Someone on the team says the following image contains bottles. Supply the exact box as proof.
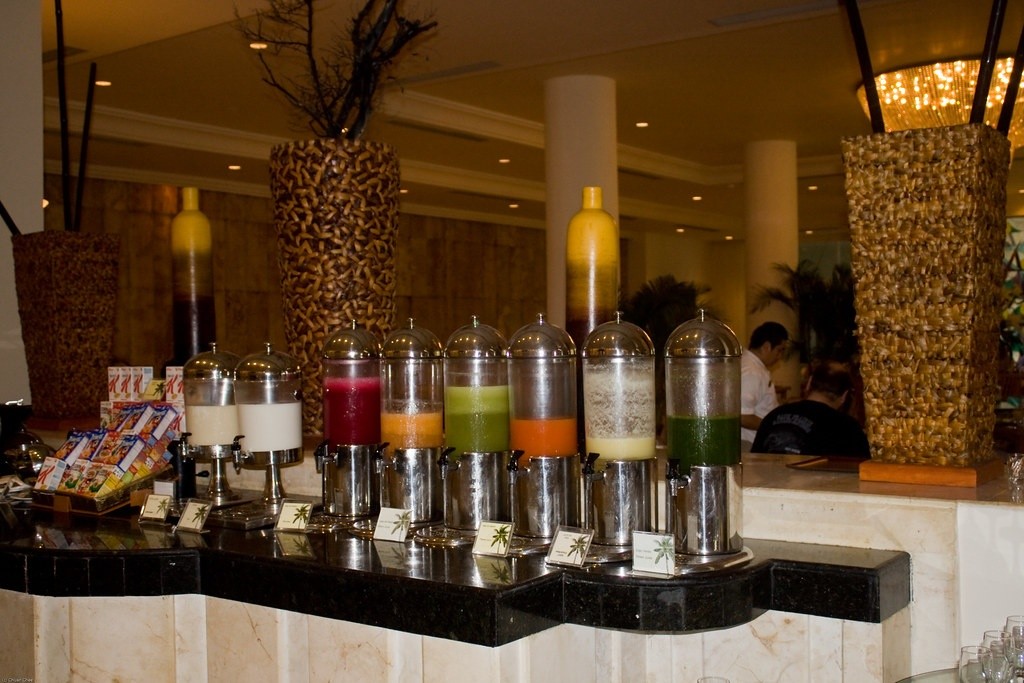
[565,186,620,466]
[170,187,215,365]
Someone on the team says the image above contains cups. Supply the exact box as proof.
[956,614,1024,683]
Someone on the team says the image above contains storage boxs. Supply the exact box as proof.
[35,367,186,497]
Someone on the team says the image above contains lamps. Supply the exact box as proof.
[855,58,1024,169]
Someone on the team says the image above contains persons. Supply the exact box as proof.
[749,358,872,458]
[740,321,789,445]
